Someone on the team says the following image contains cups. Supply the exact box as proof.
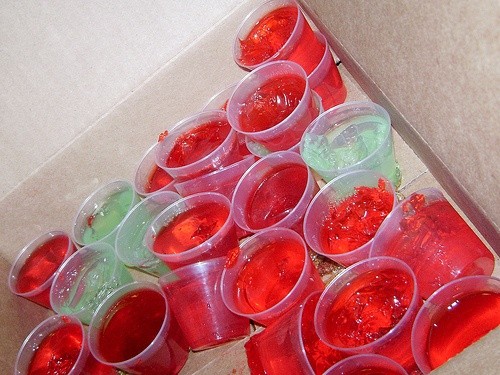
[87,281,189,375]
[13,313,119,375]
[245,88,326,159]
[49,242,137,328]
[172,154,258,238]
[224,60,319,154]
[115,189,188,282]
[231,150,324,262]
[145,193,241,280]
[321,353,410,375]
[233,0,323,79]
[131,141,182,197]
[220,229,324,328]
[367,186,495,306]
[306,30,347,113]
[153,110,244,181]
[302,169,404,268]
[411,273,500,375]
[71,180,144,257]
[155,257,248,354]
[314,255,424,375]
[197,84,246,147]
[244,292,345,375]
[7,230,85,313]
[299,99,401,194]
